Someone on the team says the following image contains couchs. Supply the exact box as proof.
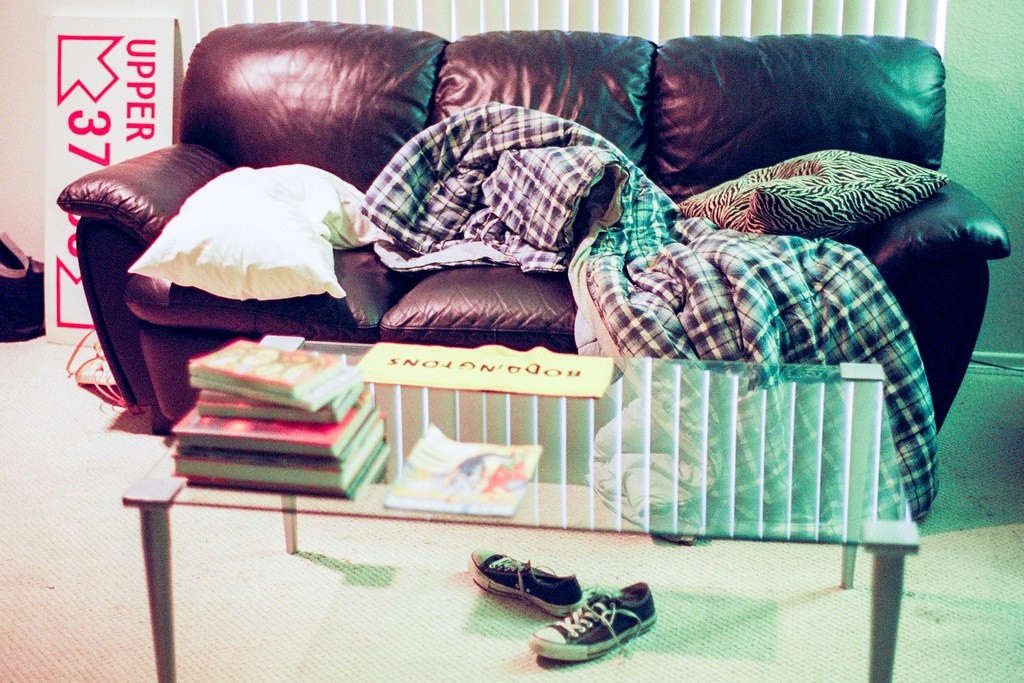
[56,20,1011,472]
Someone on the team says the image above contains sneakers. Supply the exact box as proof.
[528,582,657,661]
[467,548,583,617]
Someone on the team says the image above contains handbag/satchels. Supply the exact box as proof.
[0,232,45,342]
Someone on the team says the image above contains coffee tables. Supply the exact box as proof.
[125,334,921,681]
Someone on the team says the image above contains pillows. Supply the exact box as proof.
[679,149,949,232]
[129,163,371,302]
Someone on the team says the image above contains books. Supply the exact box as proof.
[171,338,390,502]
[383,422,544,518]
